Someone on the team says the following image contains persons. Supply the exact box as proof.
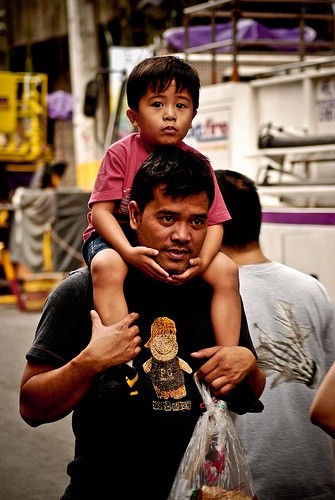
[209,170,335,499]
[310,359,335,439]
[19,145,267,500]
[81,56,265,416]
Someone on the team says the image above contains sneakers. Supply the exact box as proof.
[98,362,139,396]
[226,377,264,415]
